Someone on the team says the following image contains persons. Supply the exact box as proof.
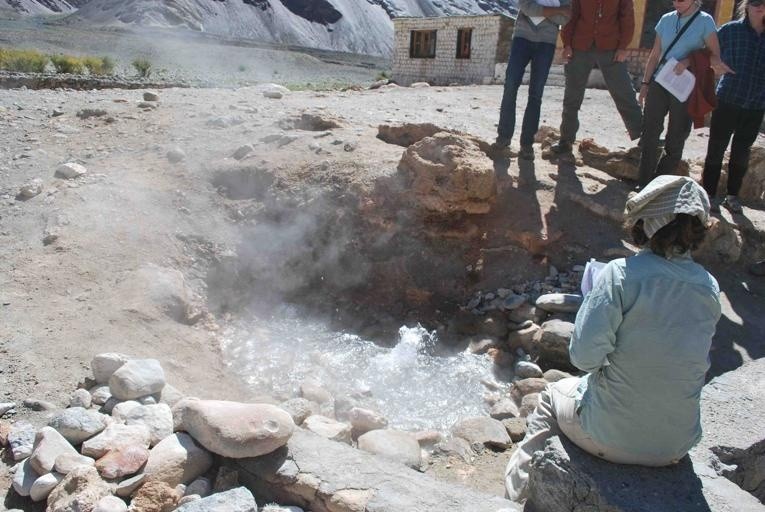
[550,1,667,153]
[504,174,724,504]
[635,1,721,193]
[699,1,764,214]
[494,1,574,159]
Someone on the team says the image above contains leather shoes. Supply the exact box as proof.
[634,184,646,191]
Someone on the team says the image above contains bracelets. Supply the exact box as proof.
[641,81,649,85]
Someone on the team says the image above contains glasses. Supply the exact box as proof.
[751,1,765,6]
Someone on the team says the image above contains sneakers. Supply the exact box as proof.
[550,141,572,152]
[496,137,510,147]
[522,134,534,144]
[710,198,720,213]
[722,198,742,215]
